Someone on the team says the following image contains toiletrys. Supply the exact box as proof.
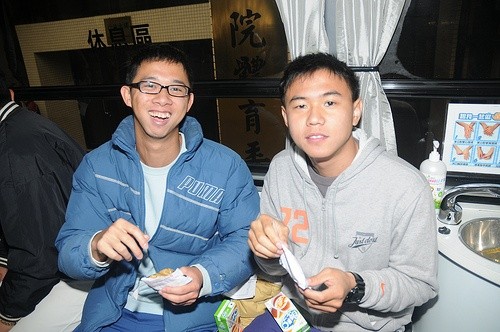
[420,140,447,215]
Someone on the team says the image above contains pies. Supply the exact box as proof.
[150,268,174,278]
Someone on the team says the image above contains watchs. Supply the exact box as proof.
[342,272,366,305]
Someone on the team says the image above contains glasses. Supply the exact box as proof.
[129,80,193,97]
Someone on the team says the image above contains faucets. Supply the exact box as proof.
[437,183,500,226]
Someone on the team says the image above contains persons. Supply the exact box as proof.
[0,78,86,331]
[243,51,439,332]
[54,42,261,332]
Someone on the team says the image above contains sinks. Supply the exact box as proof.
[438,208,500,287]
